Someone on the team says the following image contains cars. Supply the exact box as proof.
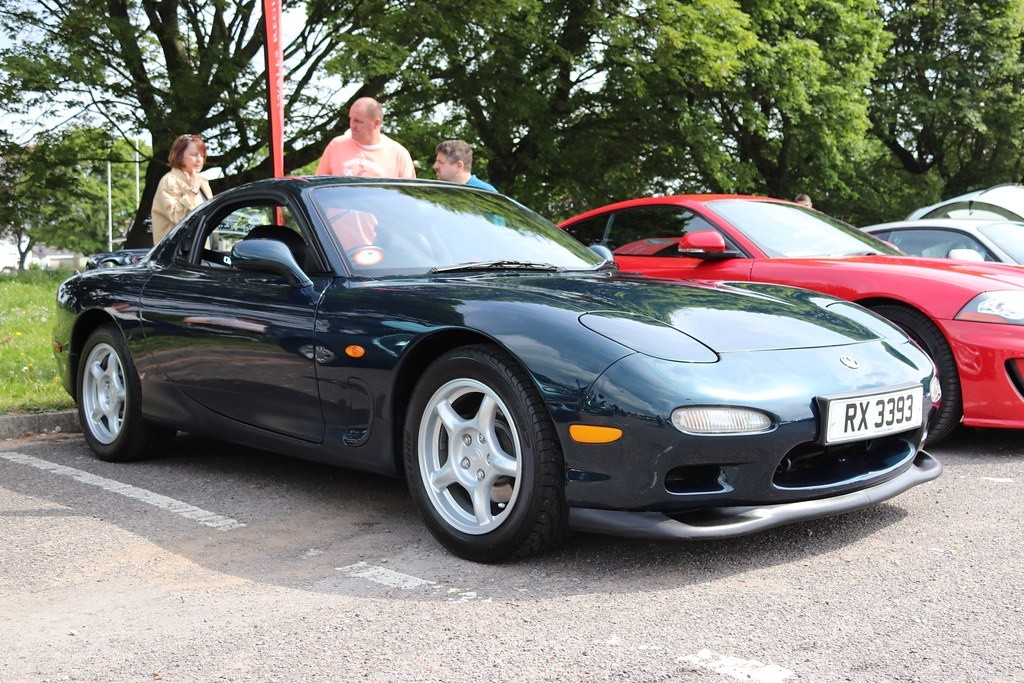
[50,176,943,566]
[555,195,1024,448]
[854,219,1024,266]
[903,184,1024,222]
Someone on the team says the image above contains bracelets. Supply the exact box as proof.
[188,186,199,195]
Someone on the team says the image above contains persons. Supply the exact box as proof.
[796,194,812,208]
[151,134,219,250]
[433,139,505,227]
[315,97,416,252]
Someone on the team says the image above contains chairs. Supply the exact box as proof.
[244,224,314,269]
[899,240,923,258]
